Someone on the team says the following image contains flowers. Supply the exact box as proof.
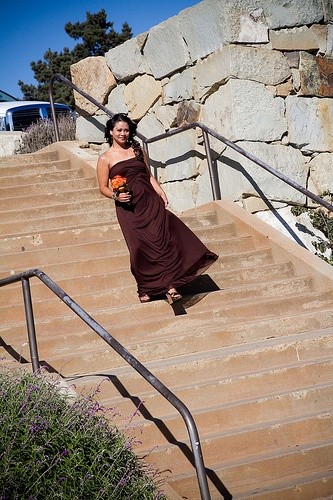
[111,175,132,207]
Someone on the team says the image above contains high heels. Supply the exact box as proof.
[140,295,150,303]
[165,292,182,304]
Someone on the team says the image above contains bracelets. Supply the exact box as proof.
[112,192,118,201]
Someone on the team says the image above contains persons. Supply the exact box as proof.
[97,113,219,304]
